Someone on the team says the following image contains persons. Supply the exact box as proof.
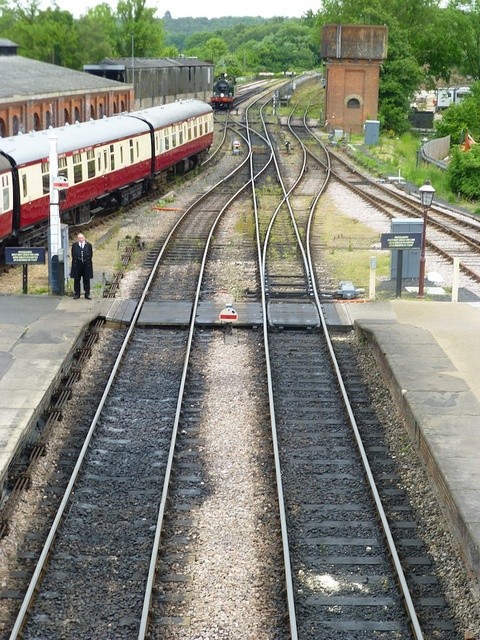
[70,234,93,300]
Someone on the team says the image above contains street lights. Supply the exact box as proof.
[418,178,435,297]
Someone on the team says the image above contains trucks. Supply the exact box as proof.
[437,91,472,111]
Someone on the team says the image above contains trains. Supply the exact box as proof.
[0,99,214,253]
[211,73,234,108]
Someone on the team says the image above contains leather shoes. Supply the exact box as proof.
[73,292,80,299]
[85,294,92,300]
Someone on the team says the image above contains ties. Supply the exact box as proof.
[80,243,83,262]
